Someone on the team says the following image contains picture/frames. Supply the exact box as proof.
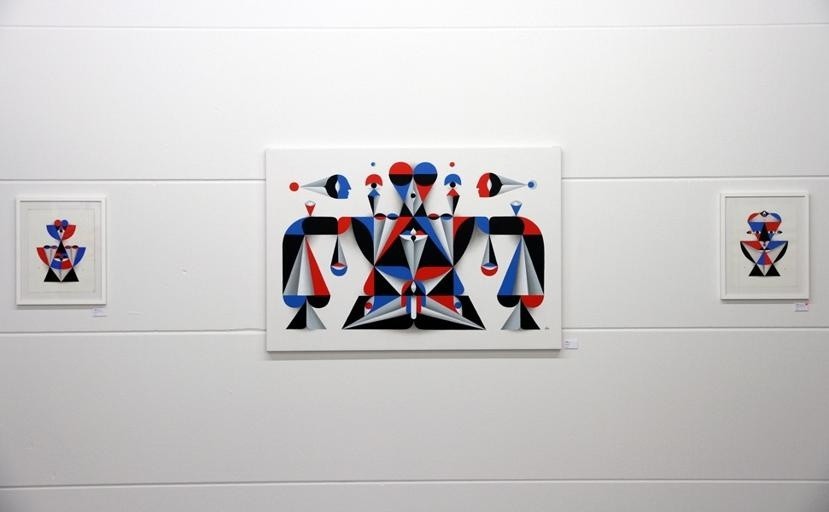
[719,192,812,302]
[16,196,108,306]
